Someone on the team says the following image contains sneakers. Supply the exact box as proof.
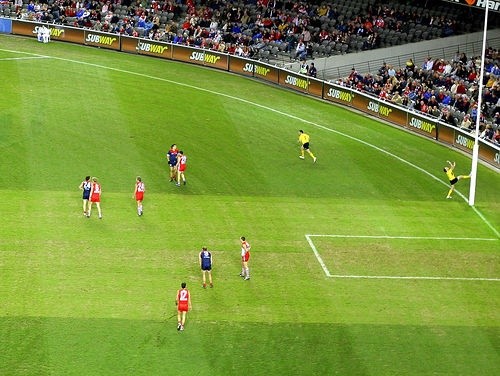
[240,274,250,282]
[299,155,305,160]
[446,196,452,199]
[168,177,187,187]
[138,210,143,216]
[177,324,184,331]
[313,157,317,164]
[82,210,101,219]
[203,283,214,291]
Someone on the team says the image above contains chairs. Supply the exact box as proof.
[0,0,500,136]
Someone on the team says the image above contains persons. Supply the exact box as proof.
[239,236,251,280]
[132,176,146,216]
[199,248,213,288]
[444,160,471,198]
[167,144,187,187]
[79,176,102,219]
[297,130,317,163]
[176,282,193,330]
[0,0,500,146]
